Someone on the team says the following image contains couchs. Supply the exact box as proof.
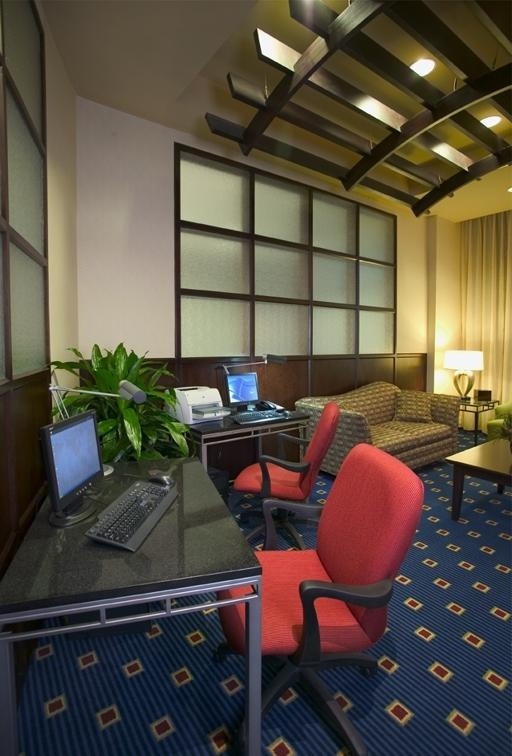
[294,380,459,479]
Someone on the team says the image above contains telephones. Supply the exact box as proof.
[255,399,286,412]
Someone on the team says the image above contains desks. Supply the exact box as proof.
[0,454,264,755]
[182,408,310,474]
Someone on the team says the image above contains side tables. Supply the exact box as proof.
[459,400,501,444]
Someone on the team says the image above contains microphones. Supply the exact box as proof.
[118,380,146,404]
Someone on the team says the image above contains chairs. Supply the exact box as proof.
[213,444,425,756]
[227,402,339,550]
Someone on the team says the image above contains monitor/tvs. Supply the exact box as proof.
[224,372,261,415]
[39,408,105,528]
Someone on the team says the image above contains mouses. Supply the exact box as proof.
[283,410,294,417]
[147,468,174,485]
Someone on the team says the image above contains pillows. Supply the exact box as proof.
[394,390,433,422]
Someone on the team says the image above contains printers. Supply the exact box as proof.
[163,386,231,425]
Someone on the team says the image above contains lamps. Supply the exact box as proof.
[443,351,485,401]
[46,380,147,476]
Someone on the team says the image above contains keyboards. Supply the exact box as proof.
[84,481,179,552]
[230,410,285,425]
